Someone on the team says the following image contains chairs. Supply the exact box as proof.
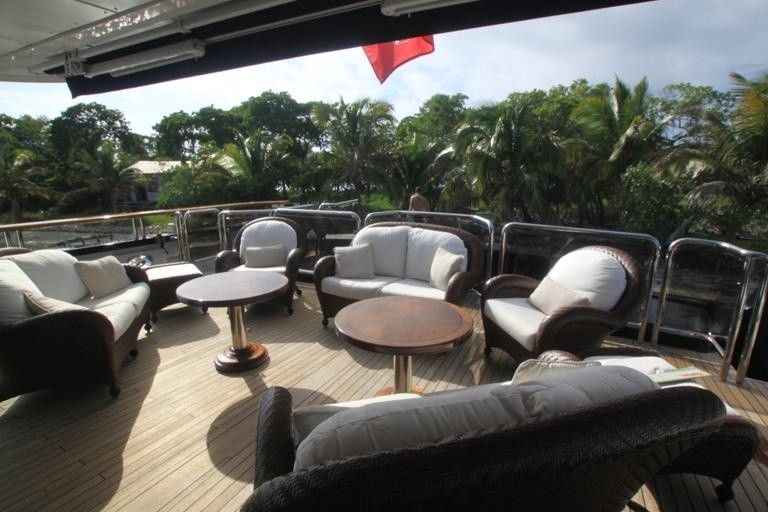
[481,245,647,365]
[215,217,306,315]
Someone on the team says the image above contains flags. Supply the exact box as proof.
[360,34,436,87]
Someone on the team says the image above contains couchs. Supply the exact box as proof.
[313,221,485,356]
[0,246,152,399]
[240,350,726,512]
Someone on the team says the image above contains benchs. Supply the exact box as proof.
[578,343,759,501]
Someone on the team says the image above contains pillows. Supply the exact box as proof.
[430,247,464,291]
[74,255,133,300]
[1,259,45,325]
[244,244,285,267]
[332,243,375,278]
[353,226,411,279]
[23,291,89,315]
[526,276,590,315]
[405,228,468,282]
[527,250,627,315]
[293,347,660,473]
[239,220,298,265]
[0,249,90,303]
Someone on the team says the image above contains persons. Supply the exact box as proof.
[408,186,426,223]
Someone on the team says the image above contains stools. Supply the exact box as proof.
[145,261,208,324]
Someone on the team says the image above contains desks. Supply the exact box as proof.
[175,271,288,372]
